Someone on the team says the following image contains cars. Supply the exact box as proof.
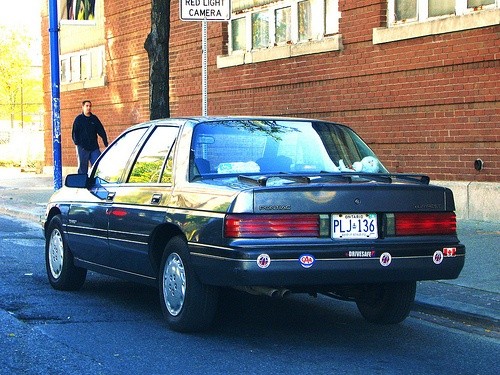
[41,114,466,334]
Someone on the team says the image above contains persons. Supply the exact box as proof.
[72,100,108,174]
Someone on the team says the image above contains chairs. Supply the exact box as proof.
[194,156,210,174]
[255,155,294,172]
